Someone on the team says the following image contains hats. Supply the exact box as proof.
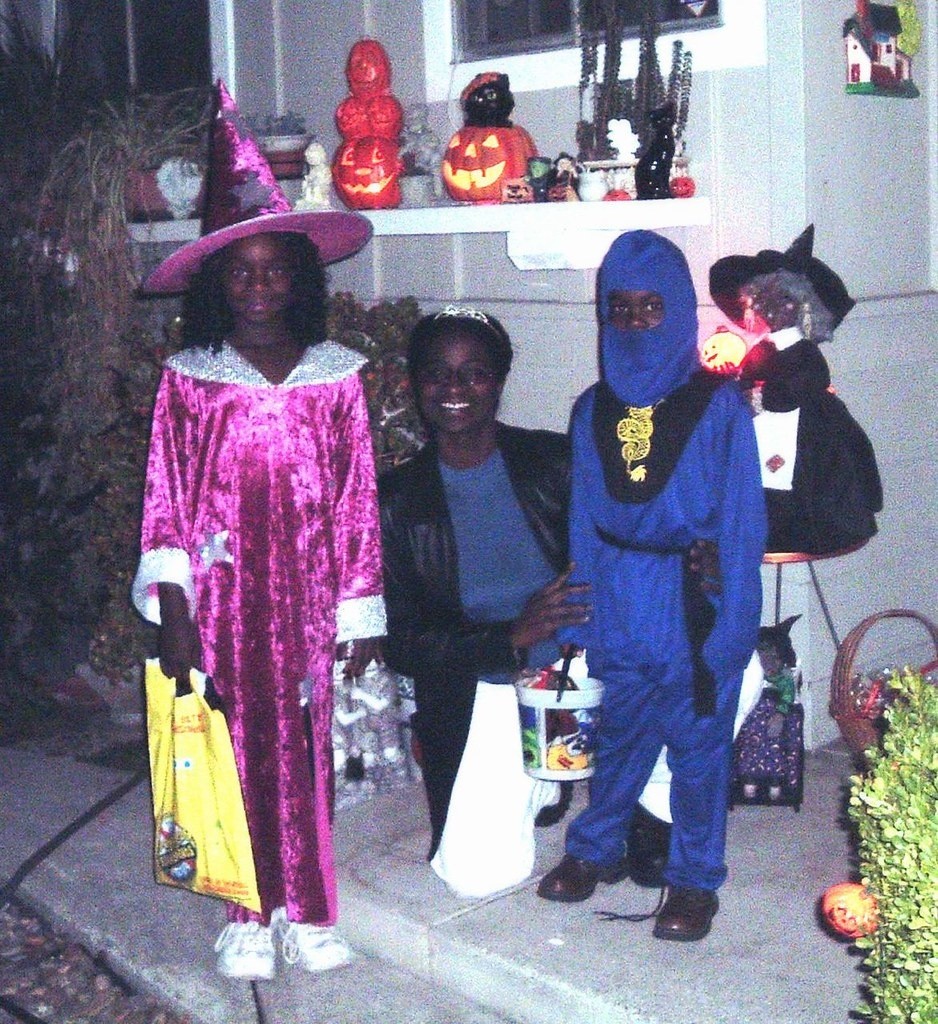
[138,80,375,294]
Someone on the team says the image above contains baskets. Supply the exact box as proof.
[830,608,938,774]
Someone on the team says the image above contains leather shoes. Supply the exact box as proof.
[537,853,625,901]
[653,885,717,937]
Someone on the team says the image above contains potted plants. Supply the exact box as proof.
[0,8,316,495]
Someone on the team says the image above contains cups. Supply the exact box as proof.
[276,179,305,208]
[265,152,309,178]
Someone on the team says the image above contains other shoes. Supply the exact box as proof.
[536,782,574,826]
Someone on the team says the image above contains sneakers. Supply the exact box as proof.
[214,920,275,981]
[276,915,355,972]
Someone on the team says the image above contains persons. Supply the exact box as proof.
[378,311,673,889]
[538,231,768,941]
[296,144,332,207]
[130,78,388,979]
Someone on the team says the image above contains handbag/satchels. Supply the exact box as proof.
[144,655,262,915]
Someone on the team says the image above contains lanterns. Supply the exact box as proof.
[442,121,536,199]
[670,176,695,199]
[331,136,401,210]
[701,326,745,372]
[822,883,879,937]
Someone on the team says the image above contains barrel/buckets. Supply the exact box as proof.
[516,647,607,781]
